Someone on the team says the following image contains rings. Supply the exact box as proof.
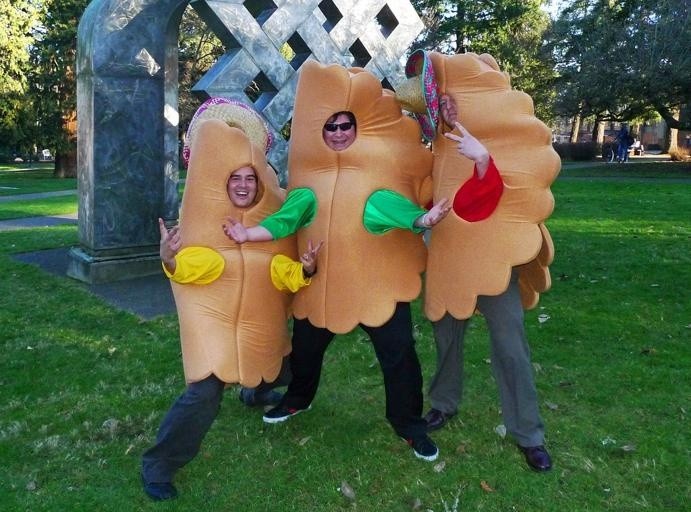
[306,255,309,260]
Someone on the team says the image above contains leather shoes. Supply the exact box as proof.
[516,441,553,472]
[420,406,458,428]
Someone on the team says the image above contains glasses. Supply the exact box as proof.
[322,121,353,131]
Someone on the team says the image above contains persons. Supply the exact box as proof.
[617,123,630,163]
[220,60,453,462]
[136,118,288,500]
[418,48,561,471]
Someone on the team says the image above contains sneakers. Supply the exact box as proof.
[141,469,179,501]
[261,401,313,424]
[238,388,284,406]
[400,432,439,462]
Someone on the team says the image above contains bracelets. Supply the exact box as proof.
[423,214,432,228]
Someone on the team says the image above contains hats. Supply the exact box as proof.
[393,47,439,143]
[182,95,272,173]
